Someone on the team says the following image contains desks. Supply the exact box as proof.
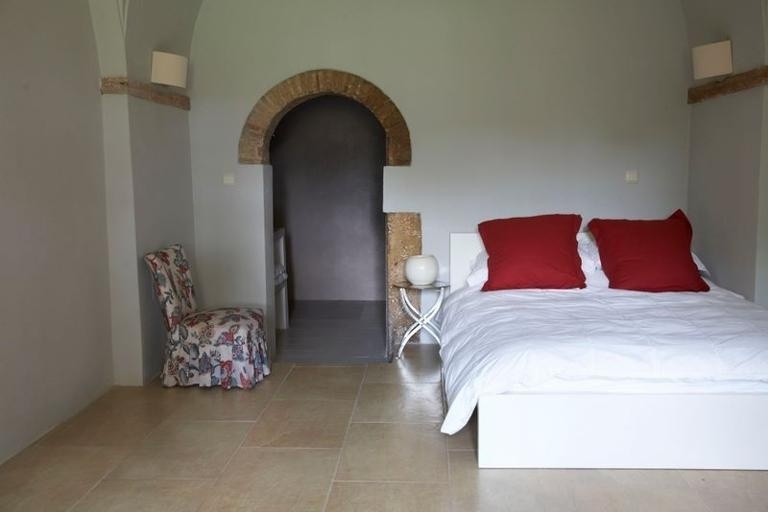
[393,281,451,361]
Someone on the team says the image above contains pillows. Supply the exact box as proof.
[466,210,711,292]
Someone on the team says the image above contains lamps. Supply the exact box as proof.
[150,51,188,89]
[692,39,733,81]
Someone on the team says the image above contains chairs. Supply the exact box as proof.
[144,244,270,389]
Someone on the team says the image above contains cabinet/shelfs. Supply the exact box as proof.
[274,227,290,331]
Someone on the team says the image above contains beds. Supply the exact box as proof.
[449,232,768,470]
